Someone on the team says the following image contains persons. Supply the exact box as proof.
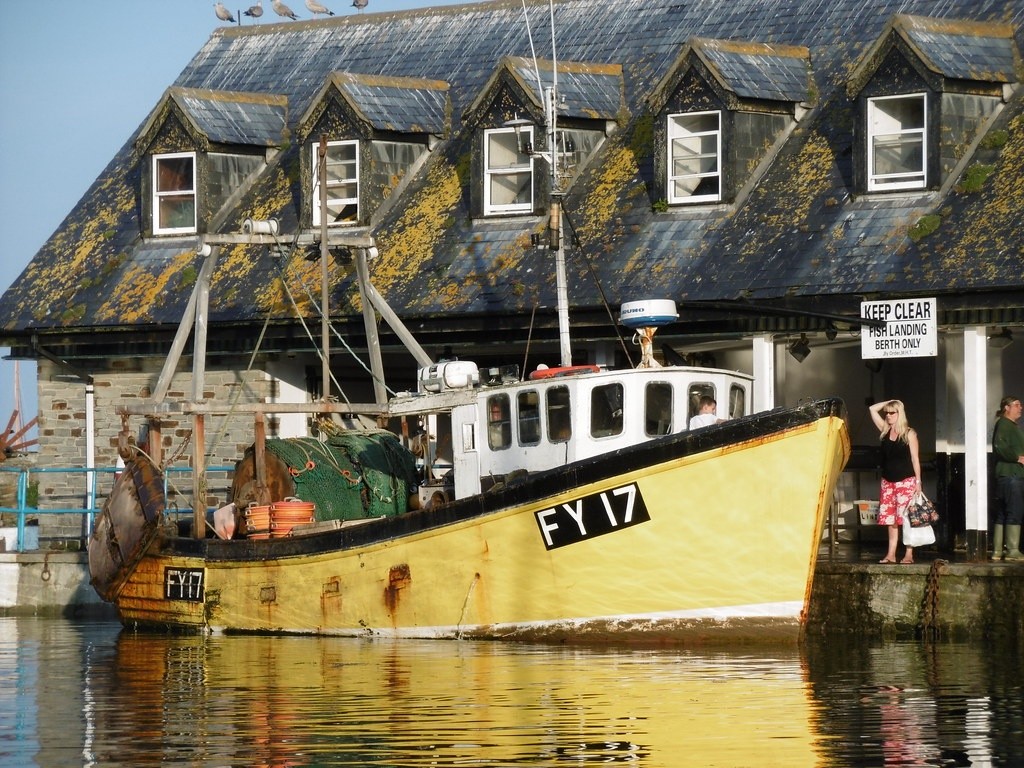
[991,396,1024,561]
[878,684,938,767]
[868,399,922,564]
[689,396,730,431]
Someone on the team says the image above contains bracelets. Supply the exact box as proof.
[916,480,922,484]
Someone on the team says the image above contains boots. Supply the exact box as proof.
[1004,525,1024,561]
[991,524,1006,560]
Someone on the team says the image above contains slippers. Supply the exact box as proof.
[878,559,897,564]
[900,559,916,564]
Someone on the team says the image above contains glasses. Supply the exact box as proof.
[885,411,898,415]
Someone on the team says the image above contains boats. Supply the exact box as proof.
[111,1,850,635]
[115,630,829,768]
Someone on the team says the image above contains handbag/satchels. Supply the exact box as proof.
[903,491,939,547]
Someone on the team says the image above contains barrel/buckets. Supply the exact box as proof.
[244,497,316,540]
